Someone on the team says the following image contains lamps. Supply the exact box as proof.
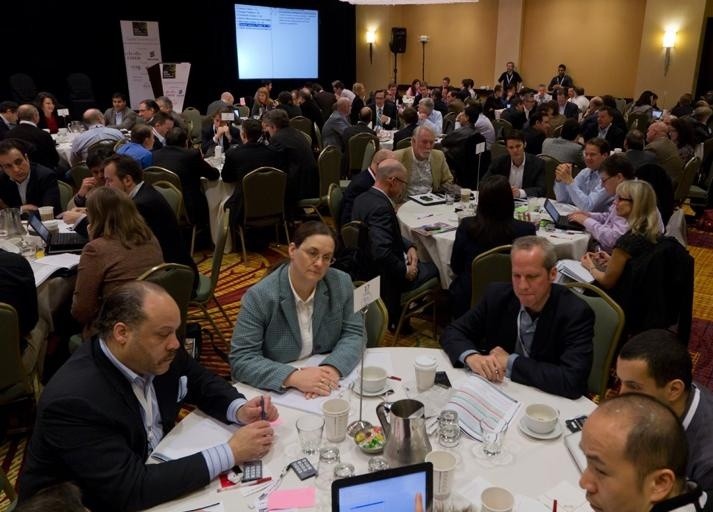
[419,35,429,83]
[662,31,674,77]
[366,32,378,65]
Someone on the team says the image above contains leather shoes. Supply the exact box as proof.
[387,322,415,337]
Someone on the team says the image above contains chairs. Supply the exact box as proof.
[564,283,622,397]
[2,304,36,502]
[473,244,516,309]
[355,282,388,347]
[66,263,199,358]
[1,98,713,257]
[192,208,235,346]
[338,220,441,345]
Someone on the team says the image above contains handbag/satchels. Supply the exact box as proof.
[180,322,231,383]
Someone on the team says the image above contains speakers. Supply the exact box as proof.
[389,27,407,53]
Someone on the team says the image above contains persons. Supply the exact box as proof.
[0,62,713,404]
[414,493,423,512]
[617,327,713,495]
[13,279,278,512]
[13,481,90,512]
[578,391,713,511]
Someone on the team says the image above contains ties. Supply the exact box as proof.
[377,109,381,127]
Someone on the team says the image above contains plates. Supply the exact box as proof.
[352,383,391,397]
[518,415,565,440]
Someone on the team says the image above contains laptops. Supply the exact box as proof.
[331,462,434,511]
[29,213,88,252]
[544,200,586,231]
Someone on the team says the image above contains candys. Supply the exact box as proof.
[357,426,387,449]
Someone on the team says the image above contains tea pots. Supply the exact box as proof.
[376,399,432,466]
[0,207,27,239]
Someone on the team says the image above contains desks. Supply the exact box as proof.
[142,346,596,509]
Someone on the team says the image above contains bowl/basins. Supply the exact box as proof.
[355,426,386,454]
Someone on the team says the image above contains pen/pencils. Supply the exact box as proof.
[553,499,557,512]
[495,370,499,374]
[550,235,573,240]
[387,376,401,381]
[217,465,290,499]
[260,395,265,420]
[417,214,434,219]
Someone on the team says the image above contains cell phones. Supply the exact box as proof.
[433,372,452,390]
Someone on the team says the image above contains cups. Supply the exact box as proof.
[368,457,389,474]
[296,415,324,454]
[322,398,351,442]
[480,415,508,456]
[37,205,54,221]
[461,188,471,203]
[21,240,37,263]
[457,208,474,224]
[358,366,387,392]
[439,410,460,448]
[413,355,440,391]
[424,450,457,500]
[480,486,514,512]
[527,196,537,211]
[525,403,561,434]
[315,447,355,491]
[445,192,455,206]
[67,121,81,133]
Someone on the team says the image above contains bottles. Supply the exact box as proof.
[215,146,220,158]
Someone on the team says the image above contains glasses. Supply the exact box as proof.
[300,247,335,266]
[601,174,613,185]
[613,194,632,204]
[389,176,409,187]
[319,447,355,479]
